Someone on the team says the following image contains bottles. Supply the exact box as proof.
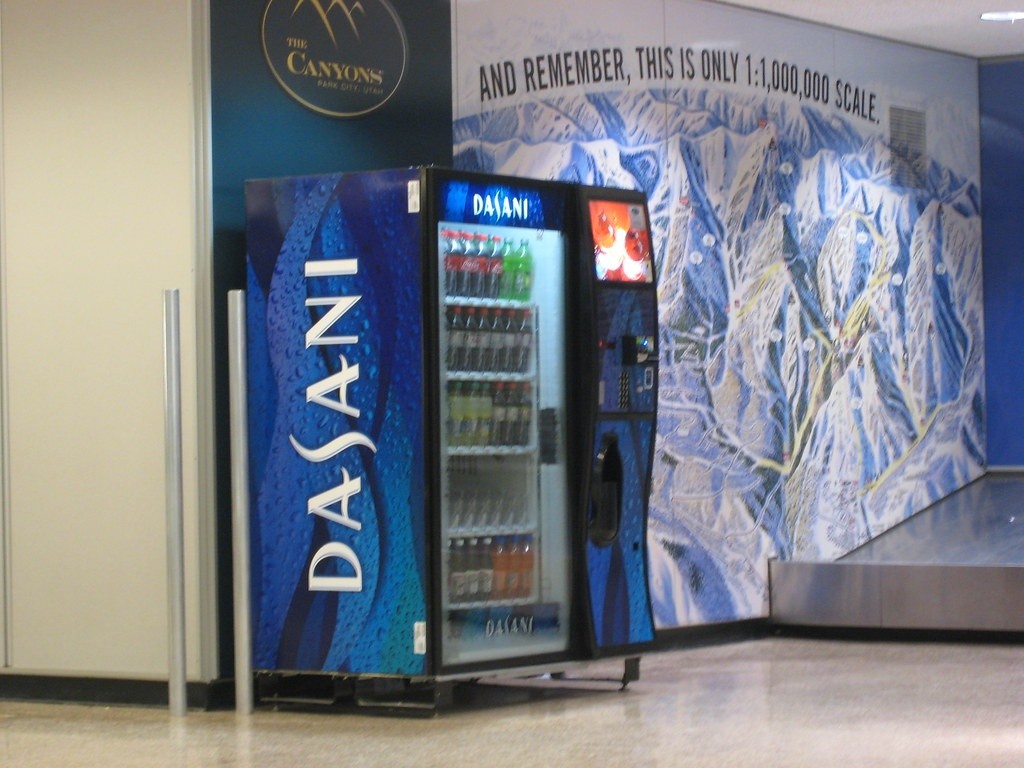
[446,306,532,373]
[448,535,536,604]
[441,228,532,302]
[448,489,530,530]
[447,380,533,446]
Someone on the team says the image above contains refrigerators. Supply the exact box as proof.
[242,164,659,718]
